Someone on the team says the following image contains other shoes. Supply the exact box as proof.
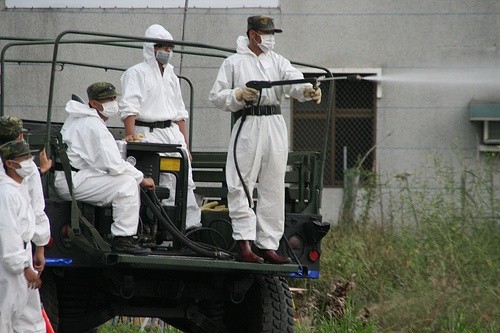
[112,236,152,256]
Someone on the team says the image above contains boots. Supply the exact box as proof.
[251,241,293,264]
[235,240,264,264]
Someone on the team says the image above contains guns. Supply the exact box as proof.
[245,75,361,107]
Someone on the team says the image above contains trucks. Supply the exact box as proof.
[0,30,336,332]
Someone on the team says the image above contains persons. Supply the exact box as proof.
[55,82,155,256]
[209,16,321,264]
[0,115,52,333]
[119,24,202,230]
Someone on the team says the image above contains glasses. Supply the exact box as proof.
[154,43,175,49]
[14,155,32,161]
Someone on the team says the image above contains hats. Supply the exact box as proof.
[87,82,122,100]
[0,140,40,160]
[247,16,283,33]
[0,115,28,135]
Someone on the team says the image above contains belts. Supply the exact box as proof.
[236,104,282,117]
[135,119,172,132]
[54,161,81,172]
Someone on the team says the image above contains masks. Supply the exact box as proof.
[249,30,275,53]
[94,100,119,117]
[155,50,173,65]
[6,156,36,179]
